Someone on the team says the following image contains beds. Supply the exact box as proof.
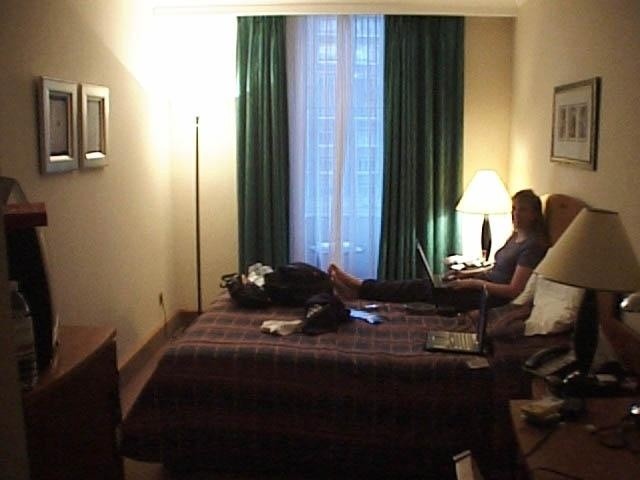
[122,194,640,466]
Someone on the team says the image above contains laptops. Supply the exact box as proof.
[424,282,488,355]
[417,241,482,292]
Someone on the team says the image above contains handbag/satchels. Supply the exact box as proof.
[220,272,264,307]
[302,295,344,334]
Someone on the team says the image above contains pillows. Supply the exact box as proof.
[510,247,553,310]
[524,276,587,340]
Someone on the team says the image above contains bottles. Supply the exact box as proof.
[9,280,40,390]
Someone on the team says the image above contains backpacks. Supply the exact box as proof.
[264,262,332,304]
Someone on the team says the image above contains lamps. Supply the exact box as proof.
[531,206,640,397]
[453,169,513,264]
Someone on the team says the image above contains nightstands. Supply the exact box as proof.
[508,398,640,479]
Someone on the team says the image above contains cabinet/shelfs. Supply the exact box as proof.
[19,326,124,480]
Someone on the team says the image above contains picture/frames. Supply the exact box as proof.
[32,75,80,177]
[549,76,601,171]
[79,82,110,169]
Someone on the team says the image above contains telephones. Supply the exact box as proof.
[520,344,578,382]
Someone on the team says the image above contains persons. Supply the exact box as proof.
[326,187,551,309]
